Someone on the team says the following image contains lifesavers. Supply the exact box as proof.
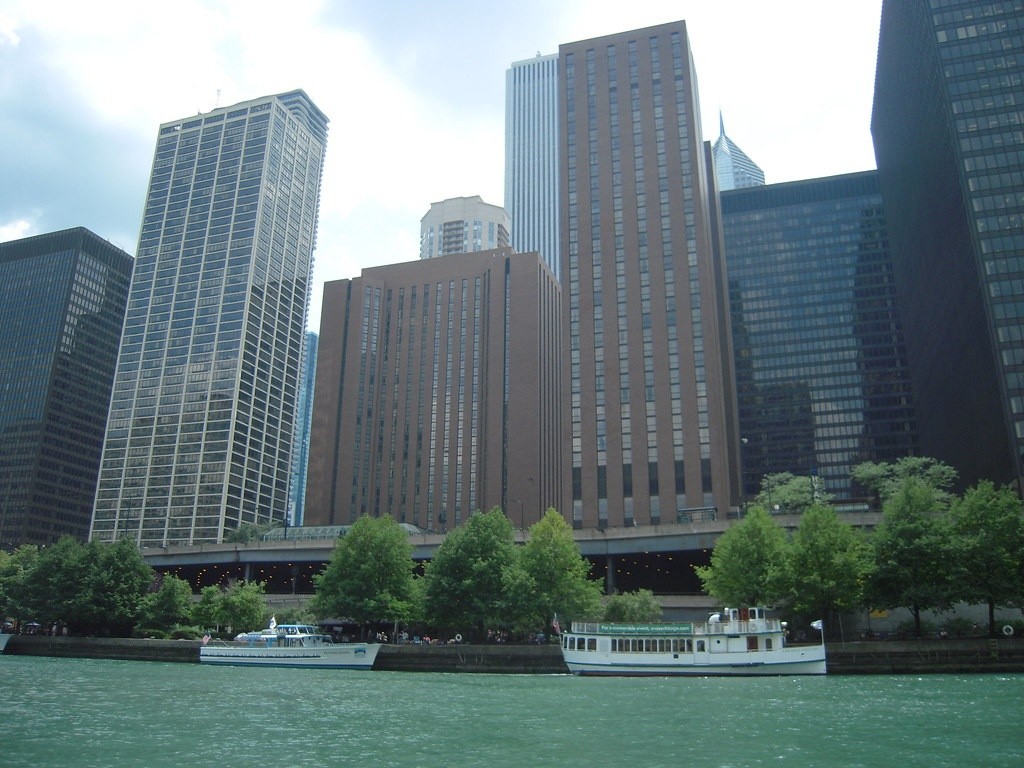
[1002,625,1013,636]
[455,634,462,641]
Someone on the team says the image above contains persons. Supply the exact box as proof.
[2,616,68,636]
[781,622,789,646]
[972,621,979,637]
[486,629,546,644]
[334,624,443,646]
[937,628,953,640]
[860,628,890,642]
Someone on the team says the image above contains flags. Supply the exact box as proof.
[552,613,560,637]
[810,620,822,630]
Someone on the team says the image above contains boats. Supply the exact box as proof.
[199,613,383,669]
[557,606,828,676]
